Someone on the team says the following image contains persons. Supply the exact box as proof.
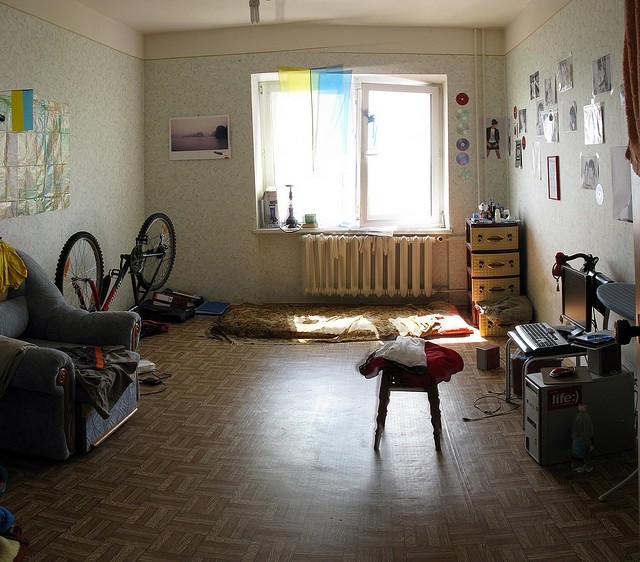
[588,159,598,189]
[529,76,538,99]
[536,103,544,136]
[568,106,576,132]
[486,118,501,159]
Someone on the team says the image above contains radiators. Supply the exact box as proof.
[300,232,436,298]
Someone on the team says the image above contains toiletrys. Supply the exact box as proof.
[495,209,501,223]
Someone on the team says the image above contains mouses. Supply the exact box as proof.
[549,367,576,378]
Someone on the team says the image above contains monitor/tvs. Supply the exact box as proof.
[556,265,591,343]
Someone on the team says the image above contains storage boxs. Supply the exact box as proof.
[474,301,529,337]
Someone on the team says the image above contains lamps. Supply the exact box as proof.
[249,0,260,24]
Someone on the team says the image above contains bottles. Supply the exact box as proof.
[495,209,500,223]
[571,404,595,472]
[489,198,494,212]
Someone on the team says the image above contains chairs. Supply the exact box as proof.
[505,266,614,430]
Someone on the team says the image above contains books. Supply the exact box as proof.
[152,300,193,312]
[193,300,231,315]
[152,291,195,307]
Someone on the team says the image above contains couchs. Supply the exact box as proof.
[0,246,142,471]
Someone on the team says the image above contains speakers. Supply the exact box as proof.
[587,344,622,377]
[476,344,500,371]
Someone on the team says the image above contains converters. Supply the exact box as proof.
[144,377,160,386]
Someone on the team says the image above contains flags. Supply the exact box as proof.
[10,89,34,132]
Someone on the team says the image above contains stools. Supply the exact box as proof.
[374,368,444,453]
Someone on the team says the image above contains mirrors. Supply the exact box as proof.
[546,155,560,200]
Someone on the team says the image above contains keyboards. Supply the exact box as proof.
[514,322,570,351]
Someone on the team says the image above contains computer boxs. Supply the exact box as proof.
[524,369,634,466]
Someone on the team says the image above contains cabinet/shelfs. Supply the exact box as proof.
[463,216,527,328]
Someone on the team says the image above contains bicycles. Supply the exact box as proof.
[53,212,176,339]
